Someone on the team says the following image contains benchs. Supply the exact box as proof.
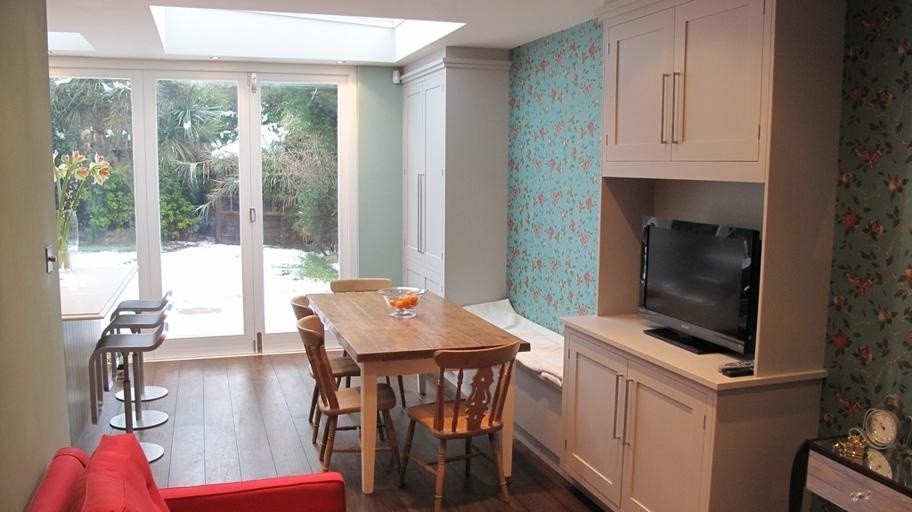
[459,298,566,474]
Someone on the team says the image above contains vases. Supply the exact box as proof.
[55,209,79,276]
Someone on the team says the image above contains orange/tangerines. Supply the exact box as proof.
[389,295,418,309]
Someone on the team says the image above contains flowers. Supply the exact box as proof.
[52,149,114,268]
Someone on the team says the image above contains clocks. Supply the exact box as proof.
[866,447,905,483]
[846,393,911,451]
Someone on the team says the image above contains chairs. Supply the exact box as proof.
[297,314,400,477]
[24,432,348,512]
[291,294,387,442]
[399,339,520,511]
[329,277,408,407]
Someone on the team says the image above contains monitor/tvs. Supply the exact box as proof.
[638,216,761,355]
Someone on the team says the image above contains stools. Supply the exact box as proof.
[88,321,170,462]
[110,290,174,402]
[101,302,173,429]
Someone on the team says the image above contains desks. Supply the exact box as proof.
[61,260,139,447]
[305,288,530,495]
[802,430,912,512]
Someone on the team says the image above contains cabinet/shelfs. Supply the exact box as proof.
[601,1,846,181]
[401,59,510,394]
[558,322,827,511]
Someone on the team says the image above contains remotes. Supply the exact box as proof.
[718,360,754,370]
[725,369,753,377]
